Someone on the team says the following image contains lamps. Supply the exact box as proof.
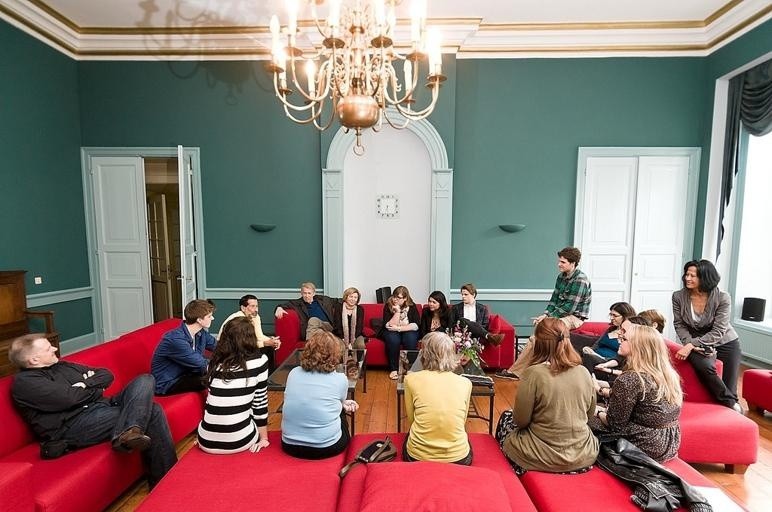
[266,0,444,158]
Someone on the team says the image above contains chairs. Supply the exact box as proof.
[0,270,61,378]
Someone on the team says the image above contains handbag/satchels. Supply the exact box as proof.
[356,440,398,463]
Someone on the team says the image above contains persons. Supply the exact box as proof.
[281,329,360,460]
[7,331,178,492]
[572,301,637,363]
[493,246,592,382]
[401,331,473,466]
[585,324,684,464]
[216,294,285,391]
[495,317,600,477]
[582,308,667,380]
[590,316,653,402]
[196,316,271,453]
[274,281,506,382]
[150,299,218,396]
[670,259,744,415]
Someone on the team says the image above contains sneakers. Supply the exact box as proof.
[389,372,399,380]
[112,427,151,454]
[486,333,504,346]
[495,369,519,381]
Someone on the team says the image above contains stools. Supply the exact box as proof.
[677,402,759,474]
[743,369,772,413]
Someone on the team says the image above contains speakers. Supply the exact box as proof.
[740,297,766,322]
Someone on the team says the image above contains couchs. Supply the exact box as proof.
[570,322,723,402]
[0,318,212,512]
[275,304,515,369]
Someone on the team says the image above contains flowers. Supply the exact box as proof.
[416,321,489,370]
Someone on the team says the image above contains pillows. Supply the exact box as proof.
[360,461,512,512]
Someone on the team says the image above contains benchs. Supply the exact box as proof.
[337,432,538,512]
[519,457,744,512]
[134,431,347,512]
